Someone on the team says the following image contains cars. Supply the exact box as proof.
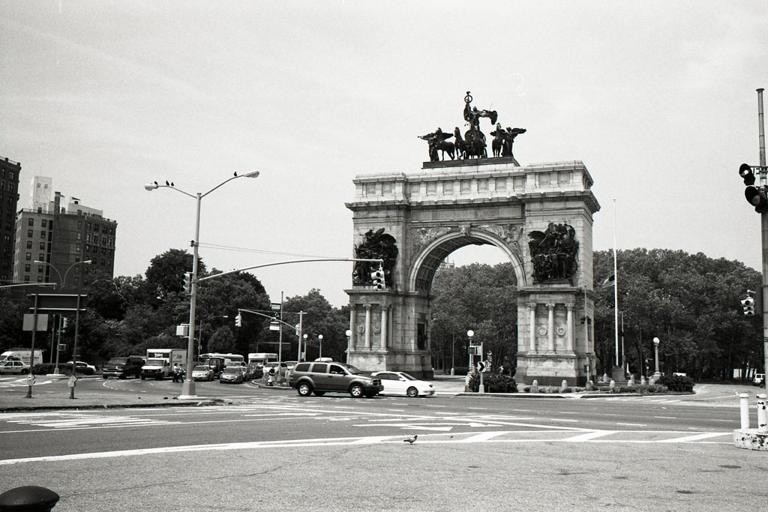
[369,371,435,398]
[0,360,31,374]
[192,352,298,384]
[66,360,96,375]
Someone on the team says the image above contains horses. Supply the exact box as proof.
[430,123,511,162]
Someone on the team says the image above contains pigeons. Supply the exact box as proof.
[404,435,418,445]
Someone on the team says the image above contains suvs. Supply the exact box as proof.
[289,361,384,398]
[102,357,145,379]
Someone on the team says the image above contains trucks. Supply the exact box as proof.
[139,348,187,380]
[0,348,47,368]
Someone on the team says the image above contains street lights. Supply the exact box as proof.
[346,330,352,364]
[144,170,259,400]
[197,315,228,363]
[303,334,308,362]
[467,329,474,377]
[34,260,91,374]
[653,337,661,380]
[318,334,323,362]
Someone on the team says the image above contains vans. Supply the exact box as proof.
[752,373,765,385]
[313,357,332,362]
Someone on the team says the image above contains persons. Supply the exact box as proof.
[173,363,179,383]
[268,365,275,386]
[178,363,184,383]
[417,102,526,163]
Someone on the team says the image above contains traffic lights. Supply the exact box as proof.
[182,273,190,295]
[235,314,241,327]
[741,297,755,318]
[376,270,385,291]
[743,184,766,214]
